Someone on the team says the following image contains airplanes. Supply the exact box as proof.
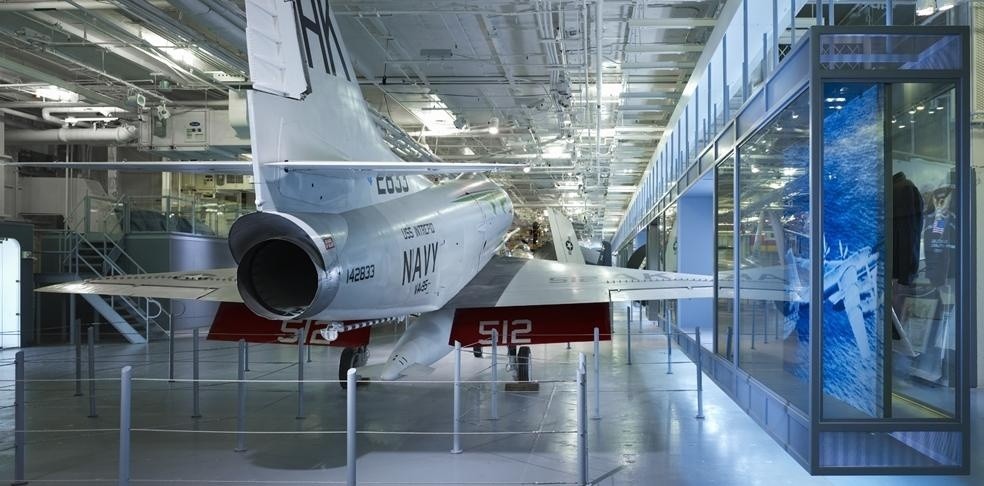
[0,0,716,388]
